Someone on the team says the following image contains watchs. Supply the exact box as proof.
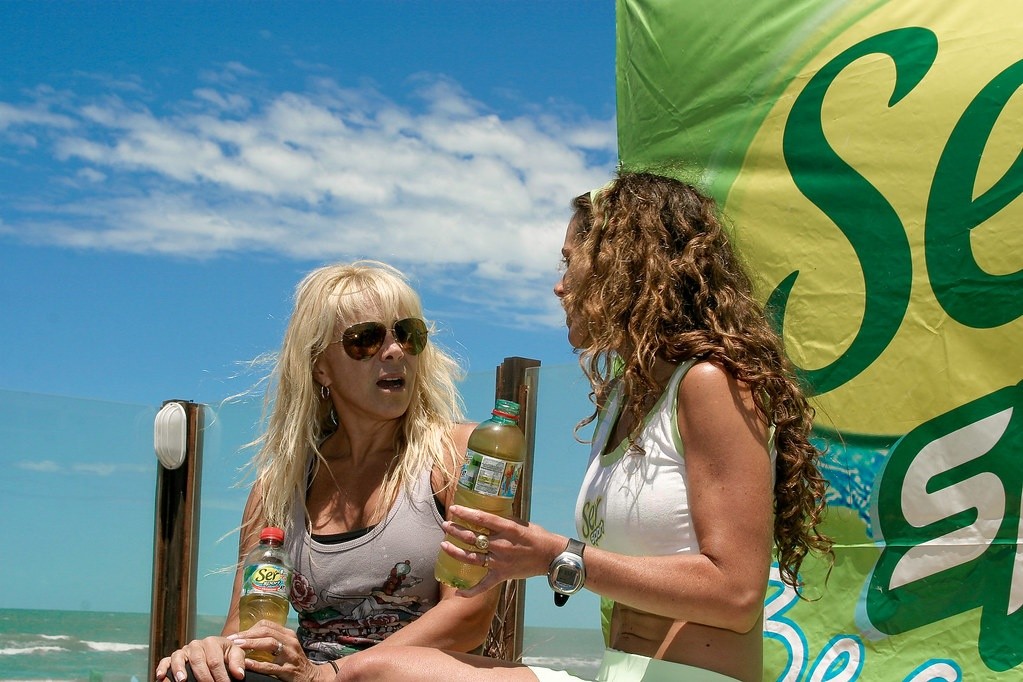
[548,538,586,607]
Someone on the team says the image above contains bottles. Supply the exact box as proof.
[238,527,293,665]
[434,399,527,590]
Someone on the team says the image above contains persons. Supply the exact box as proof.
[335,173,836,682]
[154,255,501,682]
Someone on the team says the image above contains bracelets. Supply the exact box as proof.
[327,660,339,674]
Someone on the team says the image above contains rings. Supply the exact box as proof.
[482,553,489,567]
[475,534,490,550]
[271,642,283,656]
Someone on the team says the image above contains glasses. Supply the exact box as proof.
[326,318,428,362]
